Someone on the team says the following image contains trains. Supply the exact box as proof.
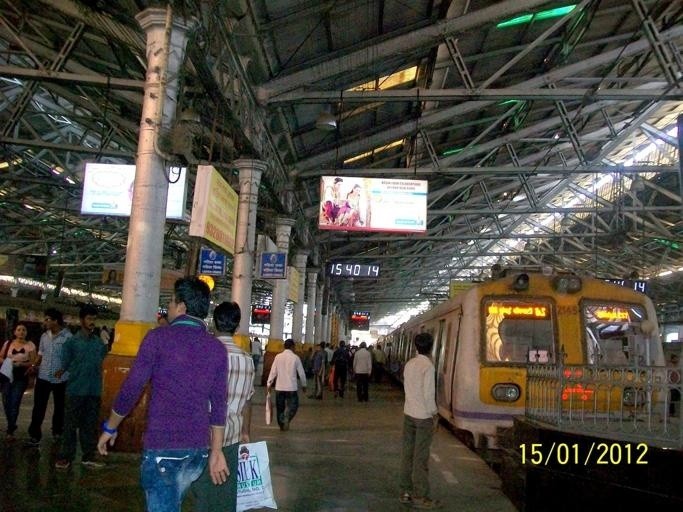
[0,294,123,342]
[370,260,671,474]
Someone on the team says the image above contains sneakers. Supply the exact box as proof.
[280,414,290,430]
[307,381,371,402]
[410,495,444,508]
[81,455,106,467]
[1,426,18,442]
[54,456,72,470]
[21,432,41,444]
[398,489,412,504]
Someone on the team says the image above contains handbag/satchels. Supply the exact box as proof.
[0,369,8,394]
[264,391,273,426]
[234,440,277,511]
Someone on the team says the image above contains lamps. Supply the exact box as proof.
[629,173,646,192]
[523,238,533,251]
[178,98,200,124]
[315,101,338,131]
[496,255,505,265]
[560,212,574,227]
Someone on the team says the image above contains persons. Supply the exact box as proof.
[322,177,344,225]
[96,276,230,511]
[338,185,366,228]
[187,301,257,512]
[399,331,446,509]
[666,355,683,420]
[2,304,119,470]
[254,331,394,428]
